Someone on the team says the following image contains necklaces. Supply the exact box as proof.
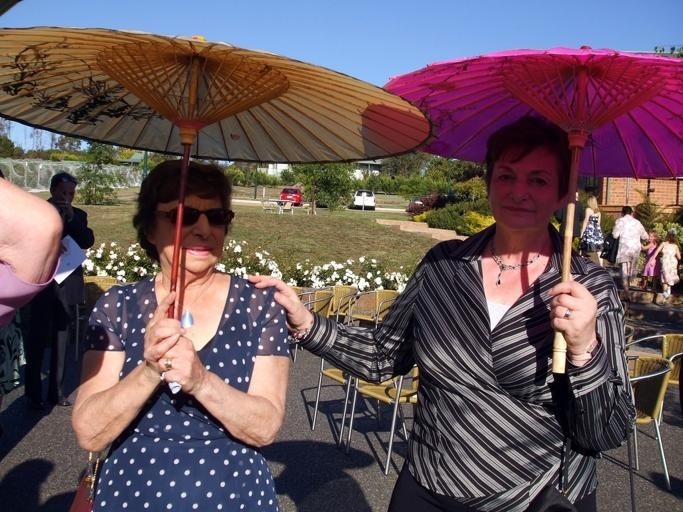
[489,234,551,289]
[159,269,224,330]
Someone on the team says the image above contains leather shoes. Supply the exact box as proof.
[55,399,70,406]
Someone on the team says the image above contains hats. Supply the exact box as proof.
[622,206,633,214]
[669,228,677,234]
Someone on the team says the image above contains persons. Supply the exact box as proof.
[245,112,641,512]
[610,204,649,302]
[18,170,96,414]
[637,229,661,292]
[70,158,301,512]
[651,231,682,306]
[0,175,65,328]
[577,193,606,269]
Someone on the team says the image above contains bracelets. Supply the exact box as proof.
[287,310,314,345]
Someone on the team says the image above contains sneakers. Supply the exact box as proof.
[666,289,671,294]
[663,292,669,299]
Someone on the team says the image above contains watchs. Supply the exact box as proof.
[567,339,600,363]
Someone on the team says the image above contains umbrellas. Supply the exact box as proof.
[381,42,683,377]
[1,22,441,339]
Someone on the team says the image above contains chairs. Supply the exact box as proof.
[622,324,683,493]
[260,196,293,215]
[63,274,120,364]
[288,282,419,474]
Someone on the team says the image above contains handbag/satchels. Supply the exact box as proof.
[70,465,97,512]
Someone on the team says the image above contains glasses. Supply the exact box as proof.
[157,207,234,226]
[59,175,77,184]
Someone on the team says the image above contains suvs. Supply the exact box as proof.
[279,187,302,206]
[352,189,375,208]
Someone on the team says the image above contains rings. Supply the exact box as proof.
[563,308,574,319]
[163,356,175,369]
